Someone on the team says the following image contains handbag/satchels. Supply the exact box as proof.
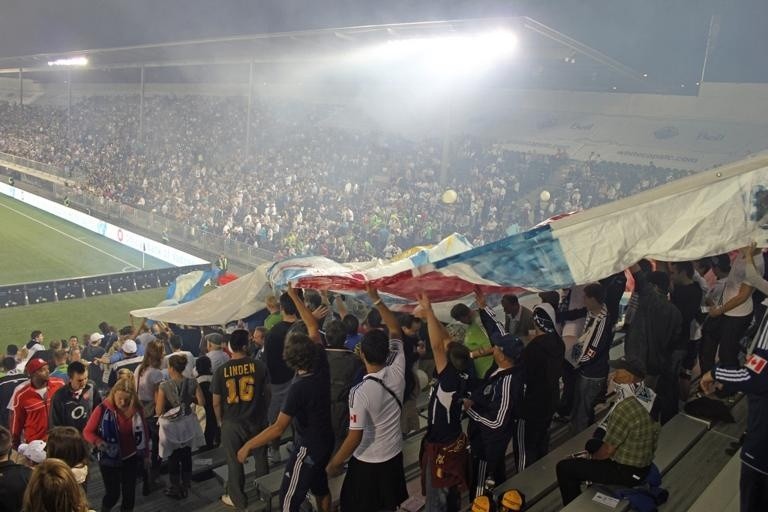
[89,425,104,462]
[143,402,157,424]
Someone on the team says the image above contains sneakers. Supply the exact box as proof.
[220,494,235,506]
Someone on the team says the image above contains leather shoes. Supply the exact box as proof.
[162,486,188,500]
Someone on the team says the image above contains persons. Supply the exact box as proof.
[0,92,768,511]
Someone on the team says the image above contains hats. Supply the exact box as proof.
[491,331,524,359]
[122,340,137,354]
[361,330,389,362]
[205,333,222,344]
[28,359,48,374]
[18,440,48,463]
[528,303,556,333]
[170,335,183,345]
[91,333,105,342]
[607,355,647,379]
